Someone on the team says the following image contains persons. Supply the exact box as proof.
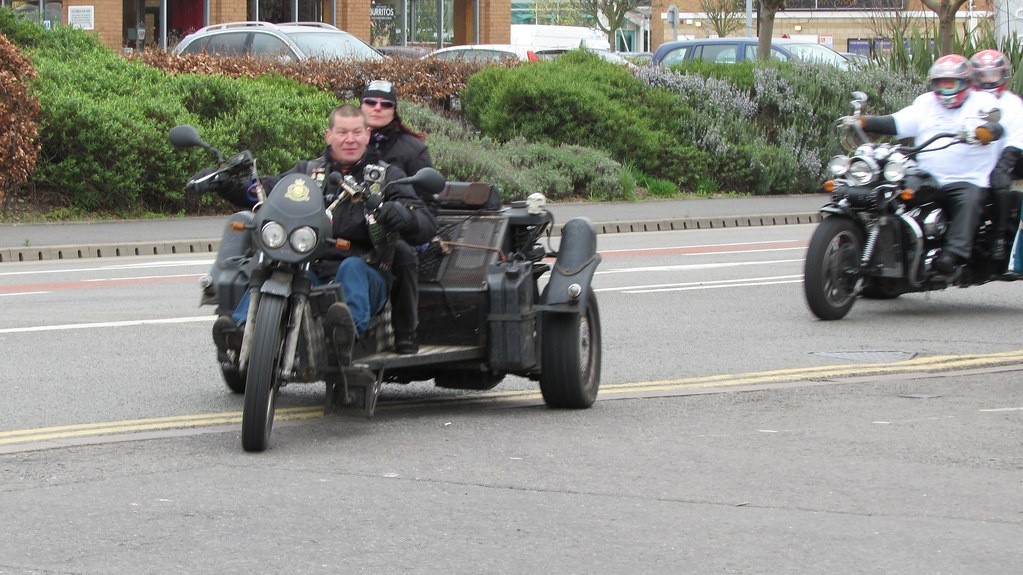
[841,53,1008,275]
[183,104,437,393]
[970,49,1023,261]
[358,79,433,354]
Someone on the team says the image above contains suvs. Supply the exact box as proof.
[164,21,393,100]
[651,36,863,74]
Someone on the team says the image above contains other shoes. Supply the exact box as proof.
[935,252,957,272]
[324,302,356,367]
[991,239,1007,260]
[212,315,238,363]
[396,332,419,353]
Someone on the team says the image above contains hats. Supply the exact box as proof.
[362,80,397,105]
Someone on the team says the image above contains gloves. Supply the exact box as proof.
[374,201,417,233]
[975,127,993,144]
[855,116,867,128]
[185,168,236,193]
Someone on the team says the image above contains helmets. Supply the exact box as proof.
[928,54,972,108]
[969,49,1011,99]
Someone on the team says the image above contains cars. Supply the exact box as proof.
[374,45,436,60]
[839,52,884,70]
[415,42,639,81]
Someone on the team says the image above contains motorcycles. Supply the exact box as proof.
[167,124,603,455]
[804,90,1023,321]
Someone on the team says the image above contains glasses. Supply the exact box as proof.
[365,100,395,107]
